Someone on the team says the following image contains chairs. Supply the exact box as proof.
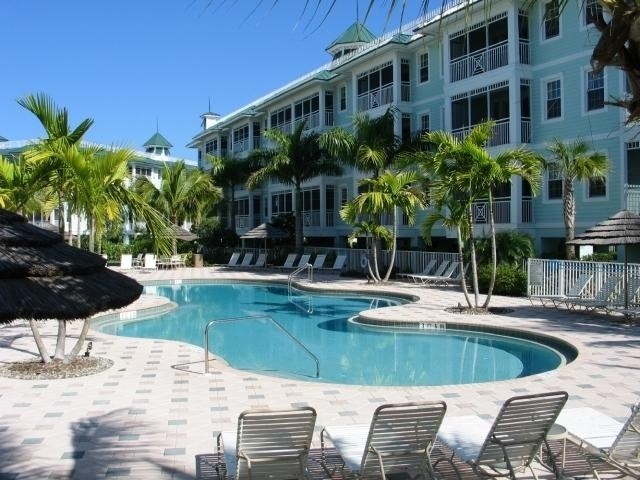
[525,270,640,324]
[215,405,317,480]
[396,257,470,288]
[109,248,348,277]
[436,390,568,480]
[320,397,446,480]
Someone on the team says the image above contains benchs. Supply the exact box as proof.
[552,387,640,479]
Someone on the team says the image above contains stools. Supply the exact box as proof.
[540,423,566,466]
